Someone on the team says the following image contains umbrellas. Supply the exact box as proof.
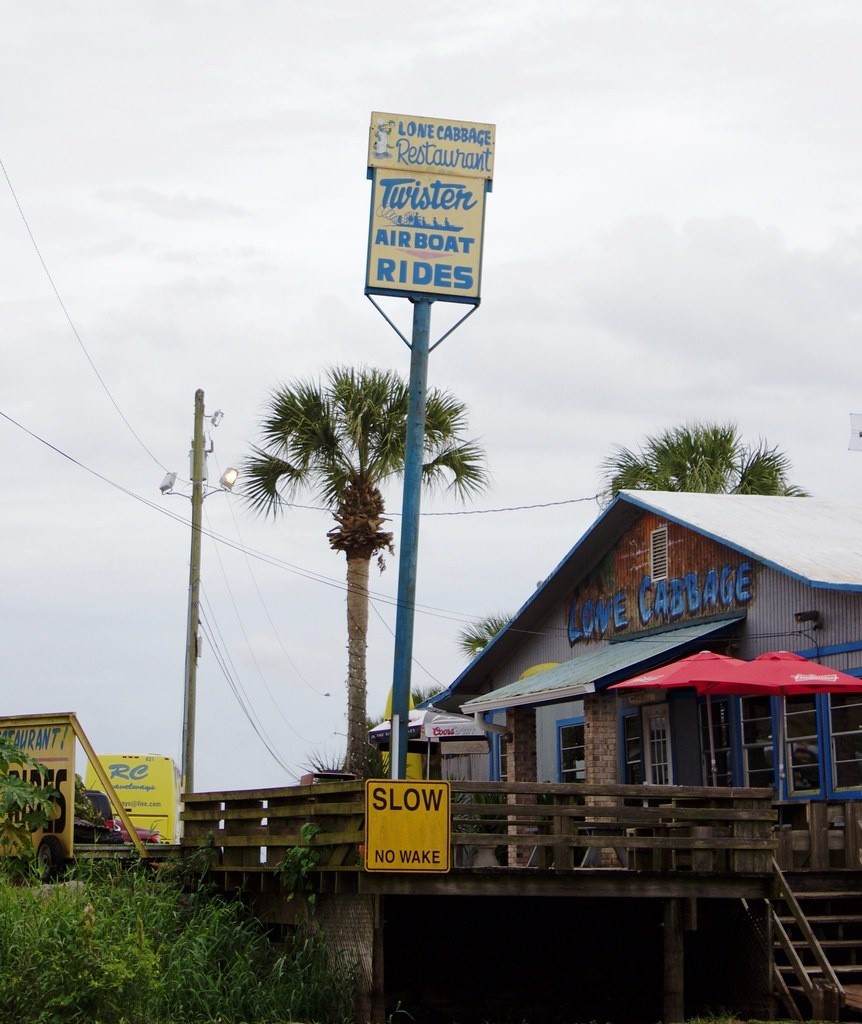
[607,650,862,801]
[368,703,491,779]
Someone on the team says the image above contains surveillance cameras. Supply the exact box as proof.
[795,609,819,624]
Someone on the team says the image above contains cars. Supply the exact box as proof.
[74,790,161,844]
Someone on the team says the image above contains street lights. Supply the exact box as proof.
[156,389,240,795]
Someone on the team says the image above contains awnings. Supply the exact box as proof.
[457,618,744,715]
